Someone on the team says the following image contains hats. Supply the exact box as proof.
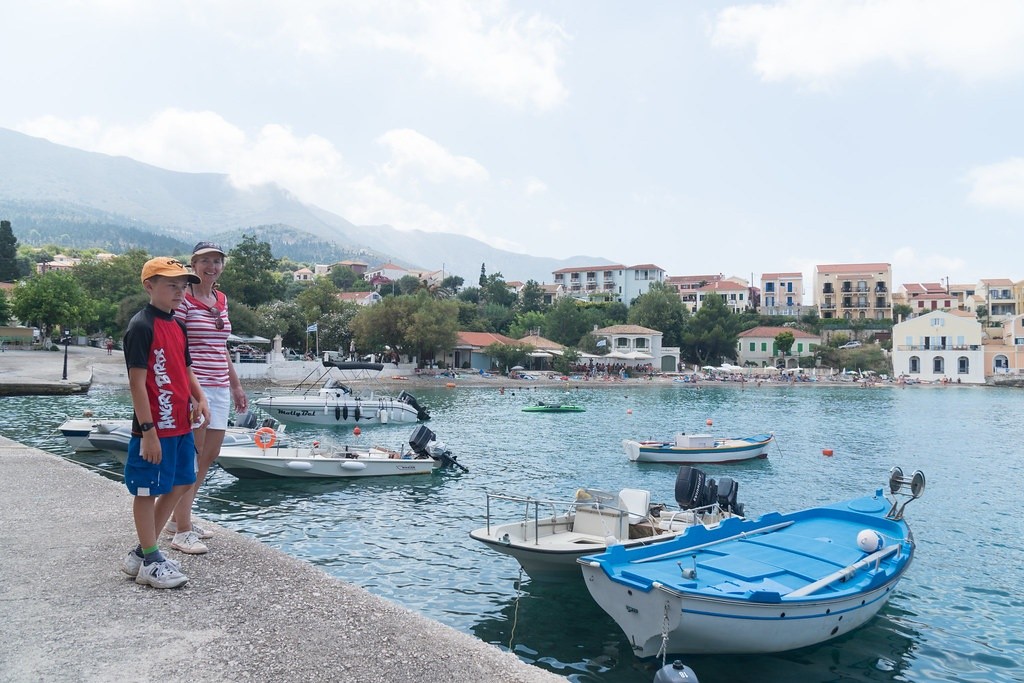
[192,241,228,258]
[141,258,201,284]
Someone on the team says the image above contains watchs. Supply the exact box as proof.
[140,422,155,431]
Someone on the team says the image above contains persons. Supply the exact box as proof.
[121,255,211,590]
[105,336,113,355]
[164,240,248,555]
[571,362,652,372]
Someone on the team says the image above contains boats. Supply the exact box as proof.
[251,350,433,424]
[0,327,96,397]
[468,465,748,584]
[266,319,424,389]
[55,407,472,478]
[576,465,927,659]
[621,430,776,463]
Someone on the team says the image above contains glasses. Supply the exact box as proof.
[210,308,224,329]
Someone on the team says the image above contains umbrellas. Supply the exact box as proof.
[511,365,524,375]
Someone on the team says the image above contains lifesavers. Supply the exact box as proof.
[256,427,276,449]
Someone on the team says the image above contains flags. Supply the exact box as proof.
[307,324,317,333]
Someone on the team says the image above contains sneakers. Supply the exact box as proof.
[122,551,181,575]
[170,530,208,553]
[163,519,214,539]
[134,560,187,588]
